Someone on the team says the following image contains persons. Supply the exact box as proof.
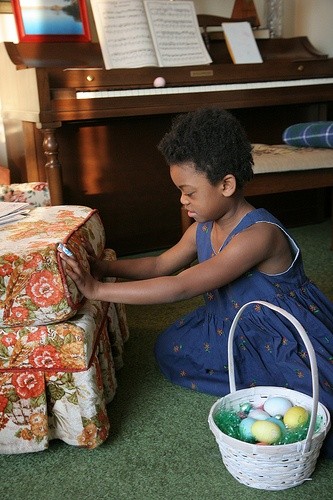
[56,107,333,423]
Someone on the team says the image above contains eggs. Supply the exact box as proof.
[239,397,309,444]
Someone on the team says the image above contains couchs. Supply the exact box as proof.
[0,182,129,454]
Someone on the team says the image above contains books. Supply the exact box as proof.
[89,0,213,71]
[222,21,264,64]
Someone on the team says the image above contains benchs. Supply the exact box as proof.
[181,144,333,234]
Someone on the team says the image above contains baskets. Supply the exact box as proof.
[208,300,330,491]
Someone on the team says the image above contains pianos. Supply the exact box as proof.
[1,35,333,256]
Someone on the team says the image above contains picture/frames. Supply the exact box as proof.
[11,0,91,43]
